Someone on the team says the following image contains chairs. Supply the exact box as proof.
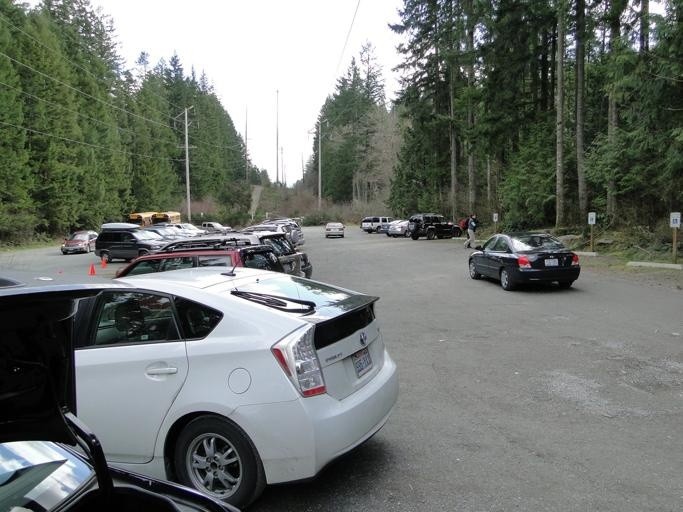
[114,298,145,342]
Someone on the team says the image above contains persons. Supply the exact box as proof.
[463,214,478,248]
[463,214,476,249]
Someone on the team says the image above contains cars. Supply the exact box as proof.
[469,232,580,290]
[362,213,461,240]
[325,222,346,238]
[61,211,313,280]
